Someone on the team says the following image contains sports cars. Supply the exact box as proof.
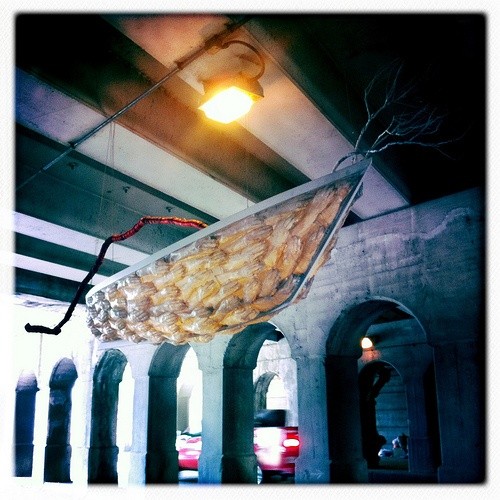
[171,424,301,483]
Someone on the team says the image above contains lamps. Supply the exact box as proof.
[195,40,265,123]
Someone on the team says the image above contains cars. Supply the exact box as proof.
[377,445,393,457]
